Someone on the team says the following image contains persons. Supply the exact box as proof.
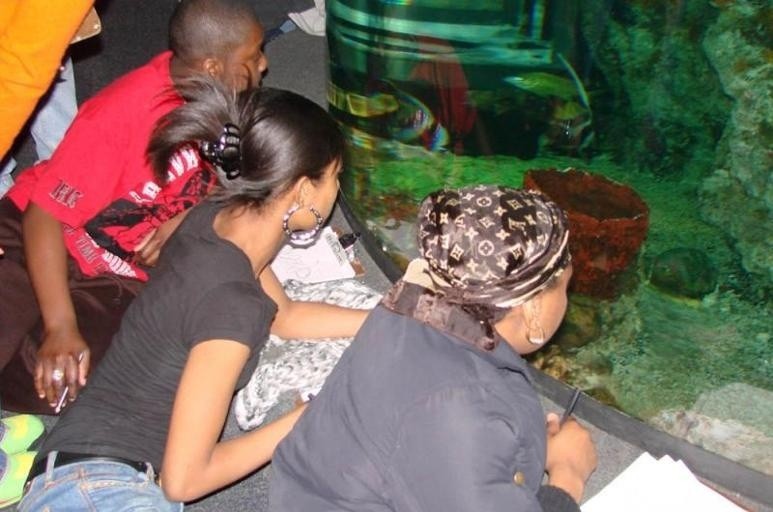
[269,184,596,512]
[15,71,372,511]
[1,0,267,414]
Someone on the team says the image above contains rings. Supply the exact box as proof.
[52,369,64,380]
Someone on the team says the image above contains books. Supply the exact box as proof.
[269,226,365,288]
[580,450,746,512]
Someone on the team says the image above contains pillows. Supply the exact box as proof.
[234,280,383,431]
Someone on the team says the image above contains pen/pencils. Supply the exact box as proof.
[560,387,581,430]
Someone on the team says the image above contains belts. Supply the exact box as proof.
[20,446,161,488]
